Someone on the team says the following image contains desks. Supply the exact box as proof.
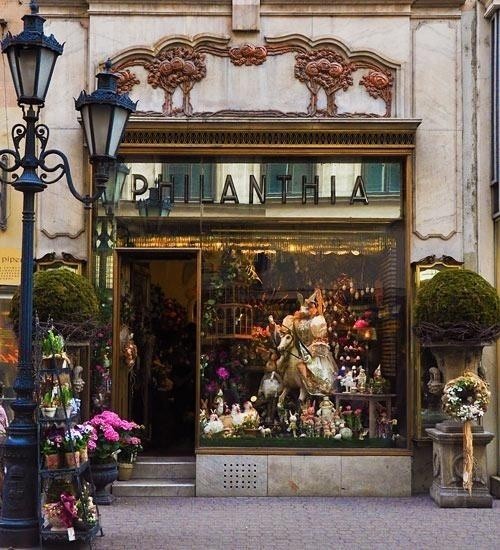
[335,391,399,440]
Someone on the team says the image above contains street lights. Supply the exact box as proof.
[1,5,141,549]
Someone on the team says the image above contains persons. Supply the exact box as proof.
[283,289,341,387]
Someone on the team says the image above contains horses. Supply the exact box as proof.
[276,328,308,419]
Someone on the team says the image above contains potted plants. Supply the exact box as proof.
[39,329,73,421]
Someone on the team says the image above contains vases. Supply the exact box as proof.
[43,445,88,469]
[88,461,134,505]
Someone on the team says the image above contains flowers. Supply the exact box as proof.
[40,494,99,534]
[84,410,145,460]
[40,424,85,453]
[440,371,492,422]
